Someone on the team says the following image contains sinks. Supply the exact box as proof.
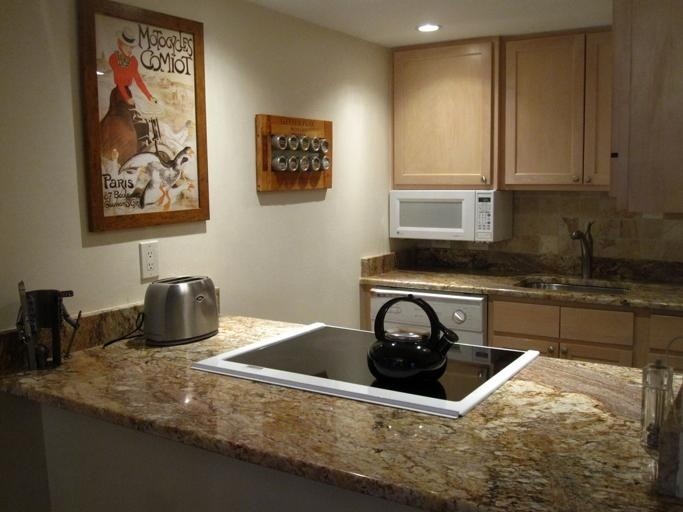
[512,273,631,296]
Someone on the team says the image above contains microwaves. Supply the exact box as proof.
[385,187,512,244]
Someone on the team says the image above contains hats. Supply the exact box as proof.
[116,30,139,46]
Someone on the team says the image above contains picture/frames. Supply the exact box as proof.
[77,1,210,232]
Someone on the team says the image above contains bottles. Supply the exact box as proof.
[639,357,673,450]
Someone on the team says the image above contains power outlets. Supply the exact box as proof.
[138,240,162,280]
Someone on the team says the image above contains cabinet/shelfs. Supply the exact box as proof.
[485,298,635,370]
[388,37,499,192]
[610,0,681,218]
[500,26,614,191]
[644,316,681,371]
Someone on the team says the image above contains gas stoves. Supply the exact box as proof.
[190,321,540,418]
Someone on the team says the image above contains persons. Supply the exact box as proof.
[99,24,159,168]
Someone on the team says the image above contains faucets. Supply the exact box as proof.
[569,219,596,278]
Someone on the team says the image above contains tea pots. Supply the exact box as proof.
[366,296,458,383]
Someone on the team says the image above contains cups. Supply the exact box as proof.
[268,132,333,174]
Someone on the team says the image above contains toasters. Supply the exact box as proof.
[143,273,220,348]
